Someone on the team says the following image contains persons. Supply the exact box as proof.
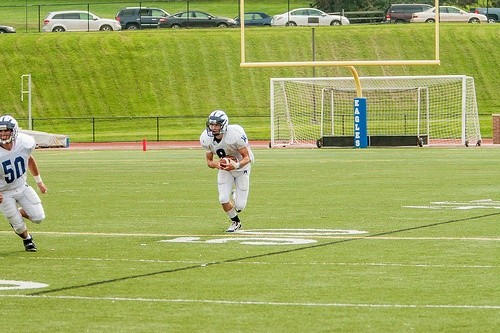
[200,110,253,232]
[0,116,47,252]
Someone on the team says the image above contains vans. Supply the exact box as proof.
[381,3,433,24]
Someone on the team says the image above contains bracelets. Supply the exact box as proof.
[34,174,42,183]
[236,163,239,169]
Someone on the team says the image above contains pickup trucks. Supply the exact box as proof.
[469,7,500,23]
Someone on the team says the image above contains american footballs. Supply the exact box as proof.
[220,155,240,169]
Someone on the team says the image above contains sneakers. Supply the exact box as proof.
[227,221,242,232]
[24,235,37,252]
[236,207,241,212]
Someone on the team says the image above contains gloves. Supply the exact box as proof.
[0,192,4,203]
[37,181,47,193]
[221,158,239,171]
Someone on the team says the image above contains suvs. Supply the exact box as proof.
[42,10,122,32]
[115,6,171,30]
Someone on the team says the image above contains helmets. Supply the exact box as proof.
[206,110,228,137]
[1,115,19,144]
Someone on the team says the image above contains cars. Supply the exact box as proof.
[157,10,238,29]
[0,24,16,34]
[409,6,488,24]
[270,7,350,26]
[233,12,272,27]
